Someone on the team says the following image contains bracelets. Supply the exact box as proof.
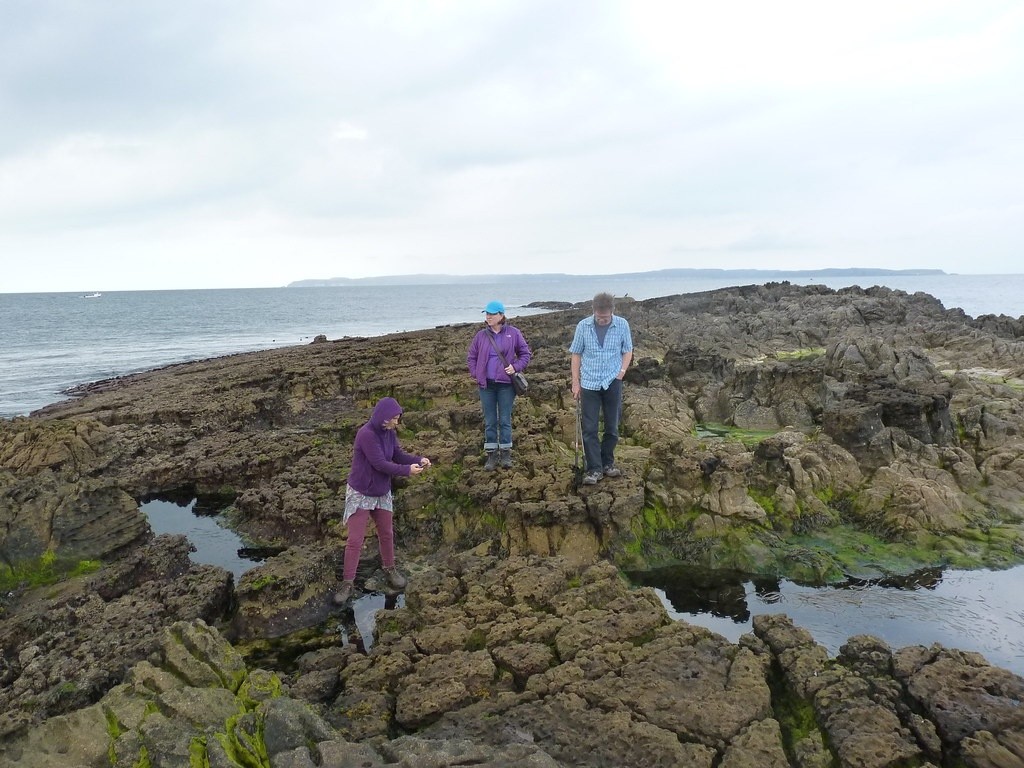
[620,369,626,374]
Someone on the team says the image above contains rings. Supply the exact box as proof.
[508,372,510,374]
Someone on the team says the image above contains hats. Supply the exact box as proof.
[482,301,504,315]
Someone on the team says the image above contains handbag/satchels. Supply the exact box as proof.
[511,373,529,396]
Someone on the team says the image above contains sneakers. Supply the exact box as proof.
[583,471,603,484]
[333,582,353,605]
[384,566,406,588]
[603,465,620,477]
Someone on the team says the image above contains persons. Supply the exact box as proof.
[467,301,531,470]
[570,293,632,484]
[333,397,432,604]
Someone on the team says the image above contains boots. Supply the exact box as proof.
[500,448,512,468]
[485,449,499,470]
